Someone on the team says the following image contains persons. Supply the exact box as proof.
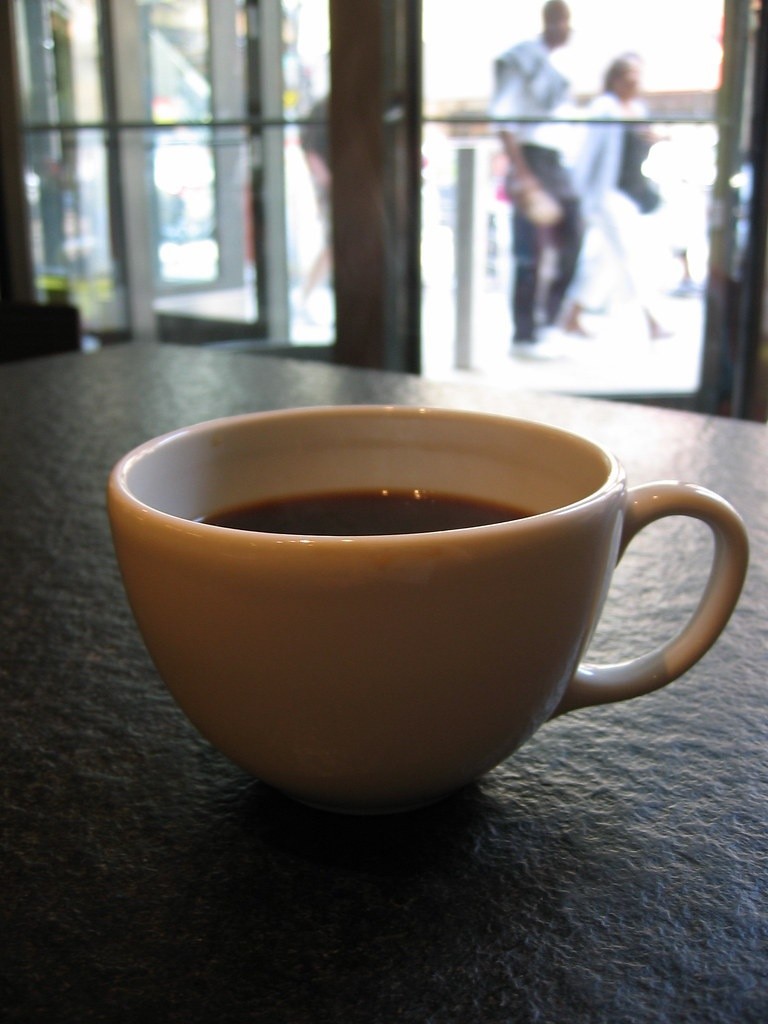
[288,94,335,333]
[485,1,705,364]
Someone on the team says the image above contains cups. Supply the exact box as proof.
[104,404,752,806]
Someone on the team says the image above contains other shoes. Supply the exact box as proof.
[512,339,553,362]
